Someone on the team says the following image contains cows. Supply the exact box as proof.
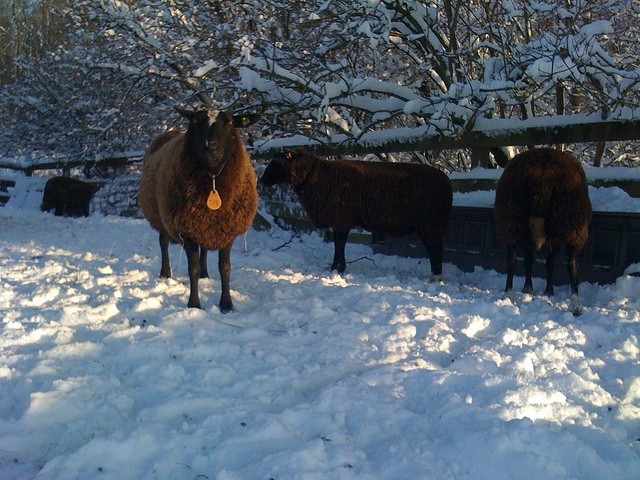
[260,150,452,279]
[494,148,592,296]
[137,98,257,314]
